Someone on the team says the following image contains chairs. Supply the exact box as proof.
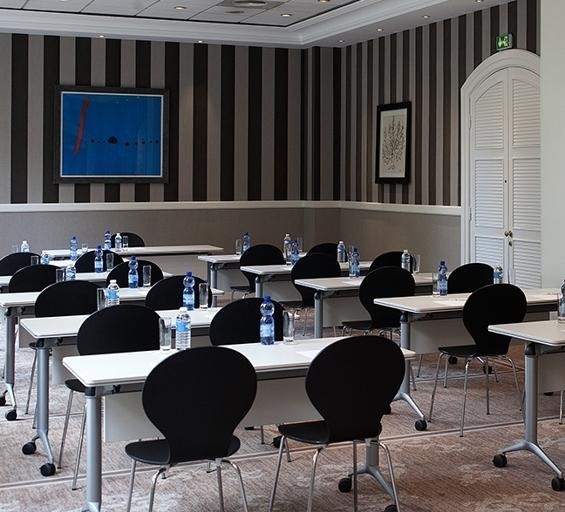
[0,233,565,512]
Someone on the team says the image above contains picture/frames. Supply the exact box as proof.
[375,101,412,184]
[53,85,169,183]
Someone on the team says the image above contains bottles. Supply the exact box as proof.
[401,249,411,272]
[258,296,275,343]
[560,280,565,315]
[243,231,361,277]
[493,263,504,284]
[11,229,195,311]
[174,308,191,350]
[437,259,447,296]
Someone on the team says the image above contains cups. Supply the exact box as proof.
[234,238,241,256]
[283,310,295,343]
[431,272,439,298]
[158,317,172,352]
[413,255,421,275]
[198,283,209,309]
